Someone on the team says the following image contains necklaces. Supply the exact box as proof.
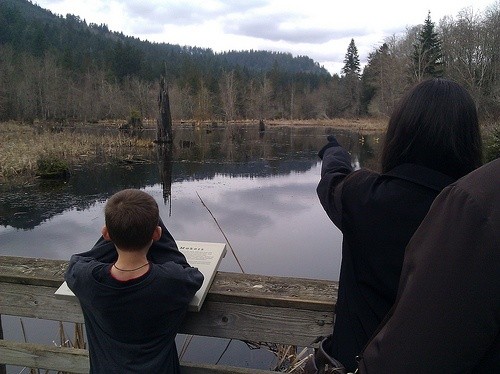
[113,262,149,272]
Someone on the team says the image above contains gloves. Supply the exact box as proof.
[317,135,341,160]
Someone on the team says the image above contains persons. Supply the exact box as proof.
[302,81,483,373]
[354,157,500,373]
[63,189,204,374]
